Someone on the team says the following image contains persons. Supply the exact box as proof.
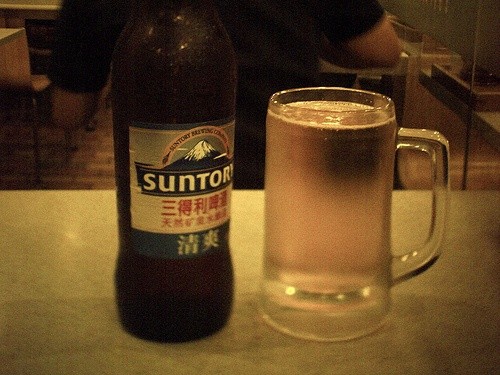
[52,0,403,192]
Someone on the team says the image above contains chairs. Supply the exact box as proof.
[7,74,76,172]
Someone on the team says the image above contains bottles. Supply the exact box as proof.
[111,0,233,343]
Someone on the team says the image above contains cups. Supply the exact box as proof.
[259,87,450,342]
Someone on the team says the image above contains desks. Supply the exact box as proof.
[0,191,500,375]
